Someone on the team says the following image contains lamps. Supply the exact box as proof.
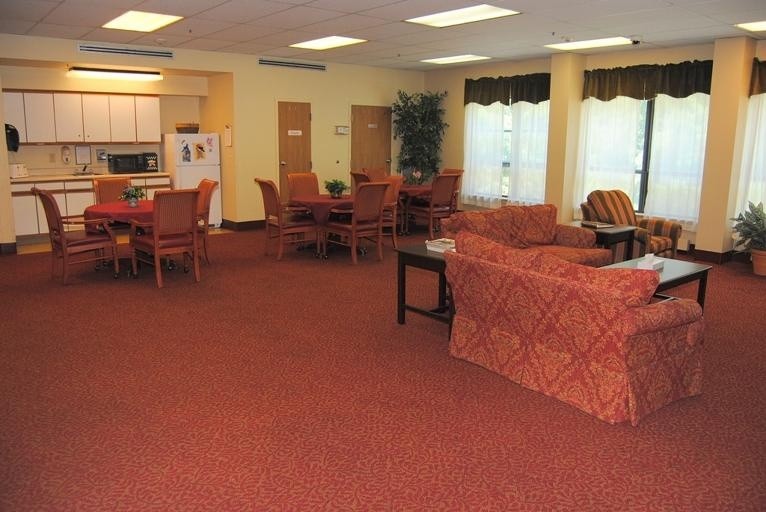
[68,67,160,81]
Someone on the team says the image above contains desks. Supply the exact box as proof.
[80,196,183,278]
[561,215,638,263]
[392,243,460,341]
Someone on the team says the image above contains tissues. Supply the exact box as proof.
[637,252,664,273]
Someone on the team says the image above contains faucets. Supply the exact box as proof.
[82,165,87,171]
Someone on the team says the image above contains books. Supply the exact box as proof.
[582,220,615,228]
[425,238,456,253]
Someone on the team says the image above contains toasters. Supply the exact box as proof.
[8,162,27,179]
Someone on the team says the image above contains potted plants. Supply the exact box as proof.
[729,199,766,276]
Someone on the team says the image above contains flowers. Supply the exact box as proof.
[116,185,144,201]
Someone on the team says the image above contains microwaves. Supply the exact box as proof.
[107,152,158,173]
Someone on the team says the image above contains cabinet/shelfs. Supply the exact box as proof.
[10,183,39,246]
[65,181,94,232]
[109,95,137,145]
[34,182,69,243]
[2,88,27,147]
[24,90,57,147]
[131,178,145,201]
[145,177,171,202]
[135,94,161,145]
[53,91,111,146]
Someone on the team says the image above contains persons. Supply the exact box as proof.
[180,139,191,162]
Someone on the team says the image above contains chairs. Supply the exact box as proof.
[163,176,219,273]
[254,165,465,267]
[94,177,136,273]
[29,186,120,281]
[580,188,683,262]
[132,186,201,289]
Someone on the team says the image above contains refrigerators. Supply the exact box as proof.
[161,132,223,228]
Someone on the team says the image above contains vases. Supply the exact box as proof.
[128,196,137,208]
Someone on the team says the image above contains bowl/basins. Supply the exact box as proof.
[176,123,200,134]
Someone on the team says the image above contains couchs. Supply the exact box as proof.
[443,231,707,423]
[439,202,615,272]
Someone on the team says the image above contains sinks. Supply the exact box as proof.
[72,173,104,176]
